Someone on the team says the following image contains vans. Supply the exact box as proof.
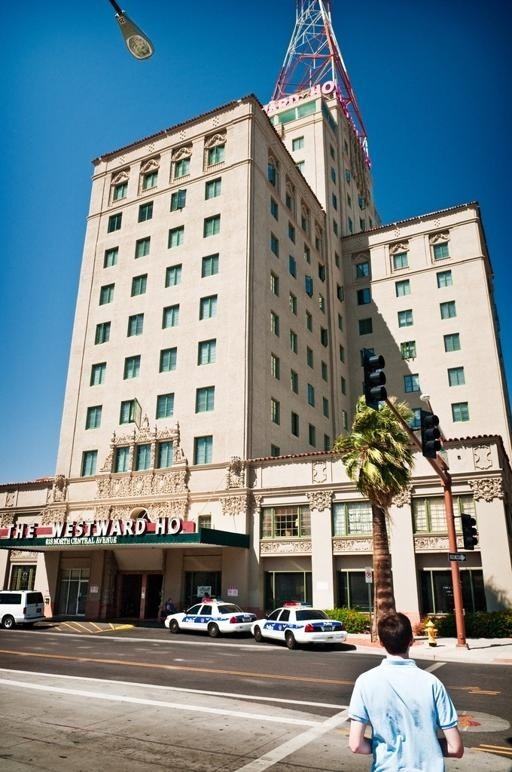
[0,590,45,630]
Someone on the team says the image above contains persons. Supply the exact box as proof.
[201,591,210,602]
[165,597,177,615]
[347,612,464,772]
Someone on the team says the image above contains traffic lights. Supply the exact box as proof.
[420,407,442,459]
[362,346,388,412]
[461,512,478,550]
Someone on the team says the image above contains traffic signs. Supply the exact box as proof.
[448,552,467,562]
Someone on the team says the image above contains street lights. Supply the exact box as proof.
[107,0,155,60]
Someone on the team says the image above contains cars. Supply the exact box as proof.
[164,598,258,638]
[250,602,348,652]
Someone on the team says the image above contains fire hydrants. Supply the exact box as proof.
[423,620,439,647]
